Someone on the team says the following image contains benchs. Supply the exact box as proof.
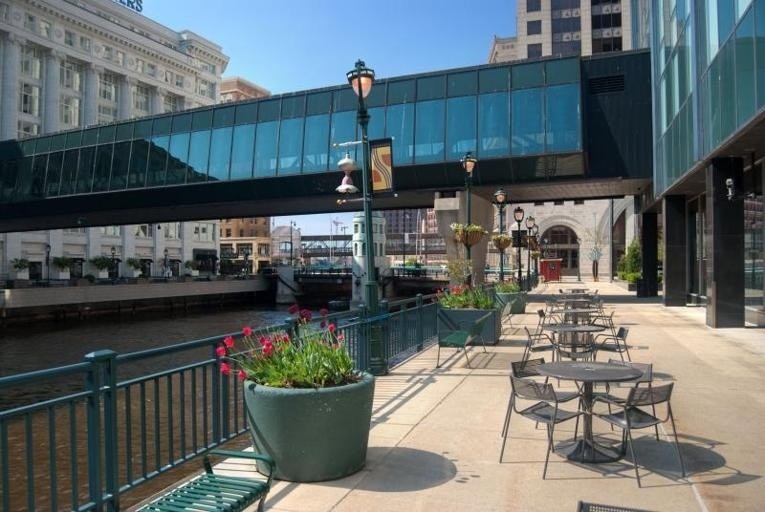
[435,310,493,370]
[123,445,273,512]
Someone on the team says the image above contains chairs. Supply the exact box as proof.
[495,287,689,485]
[578,499,658,512]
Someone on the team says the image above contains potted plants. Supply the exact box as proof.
[493,233,513,249]
[450,223,487,246]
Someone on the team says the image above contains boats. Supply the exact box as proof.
[327,294,352,309]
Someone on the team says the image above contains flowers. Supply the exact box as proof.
[215,305,356,393]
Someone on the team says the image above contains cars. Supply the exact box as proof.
[257,262,283,274]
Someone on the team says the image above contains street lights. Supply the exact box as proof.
[111,244,117,278]
[513,206,525,291]
[493,185,509,281]
[332,216,343,269]
[402,211,411,267]
[576,237,582,281]
[243,243,251,276]
[45,243,52,280]
[459,150,478,288]
[290,219,297,267]
[333,58,390,375]
[525,216,536,292]
[340,226,348,268]
[163,247,169,278]
[533,225,551,291]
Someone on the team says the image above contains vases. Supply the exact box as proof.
[239,367,376,482]
